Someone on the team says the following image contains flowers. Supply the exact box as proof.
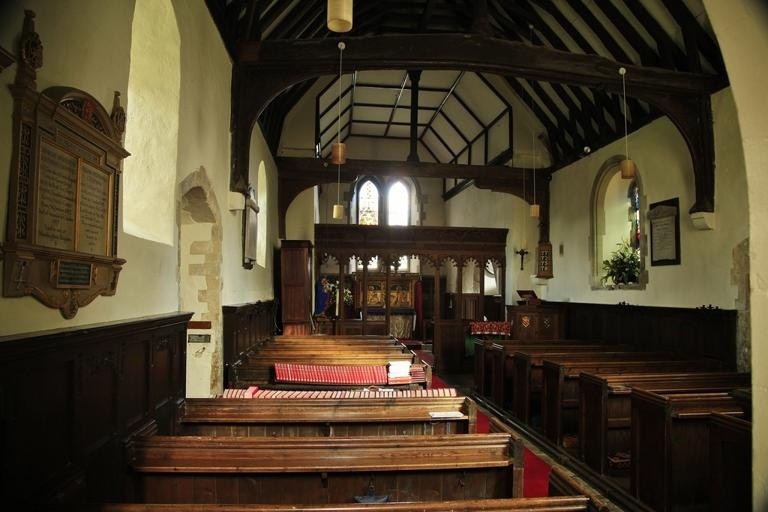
[602,238,644,288]
[320,277,353,307]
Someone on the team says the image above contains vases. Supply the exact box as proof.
[614,268,636,283]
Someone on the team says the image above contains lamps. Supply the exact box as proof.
[617,66,637,181]
[528,106,541,218]
[332,30,348,164]
[326,0,356,32]
[332,161,345,220]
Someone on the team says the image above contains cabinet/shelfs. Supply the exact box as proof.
[354,268,422,339]
[281,240,314,322]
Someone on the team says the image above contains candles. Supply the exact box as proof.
[335,289,339,316]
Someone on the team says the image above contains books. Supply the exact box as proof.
[221,357,456,400]
[428,410,466,420]
